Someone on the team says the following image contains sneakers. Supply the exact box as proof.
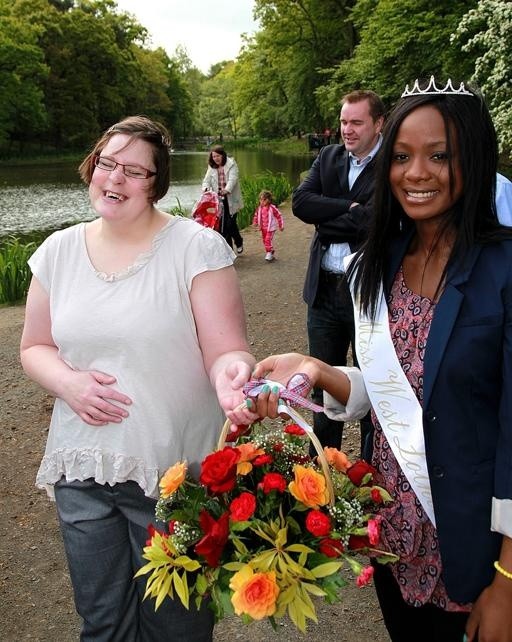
[265,252,272,260]
[237,245,243,253]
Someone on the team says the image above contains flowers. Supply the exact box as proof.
[130,418,404,637]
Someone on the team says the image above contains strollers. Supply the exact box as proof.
[190,187,225,235]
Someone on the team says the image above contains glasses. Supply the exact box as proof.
[94,156,156,179]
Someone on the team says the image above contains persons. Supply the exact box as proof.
[202,144,244,254]
[252,190,285,260]
[20,115,255,642]
